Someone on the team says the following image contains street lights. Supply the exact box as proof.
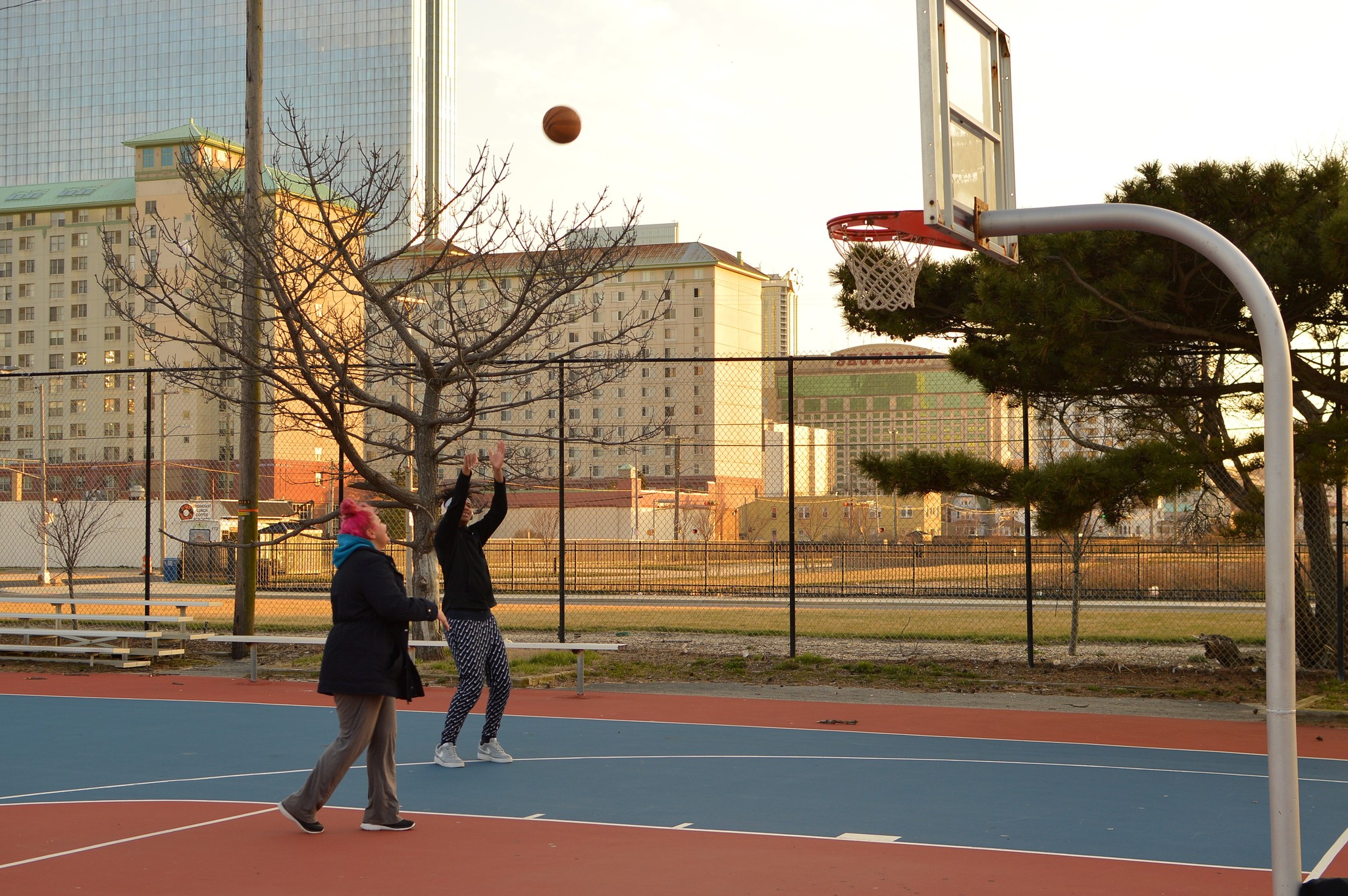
[159,424,195,574]
[395,294,427,628]
[675,467,701,541]
[322,486,333,537]
[1,365,48,586]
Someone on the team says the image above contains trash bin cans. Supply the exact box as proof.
[913,544,926,558]
[162,557,182,582]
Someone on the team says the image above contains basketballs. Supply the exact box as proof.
[542,106,582,144]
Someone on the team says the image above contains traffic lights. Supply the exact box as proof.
[878,526,885,532]
[1033,515,1037,519]
[1101,514,1106,519]
[704,501,715,506]
[843,502,855,506]
[692,528,698,533]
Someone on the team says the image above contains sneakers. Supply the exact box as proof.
[434,742,464,768]
[477,737,513,763]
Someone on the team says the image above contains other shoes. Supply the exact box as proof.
[278,801,325,834]
[361,818,415,831]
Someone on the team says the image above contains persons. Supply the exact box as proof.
[435,440,514,767]
[278,499,449,833]
